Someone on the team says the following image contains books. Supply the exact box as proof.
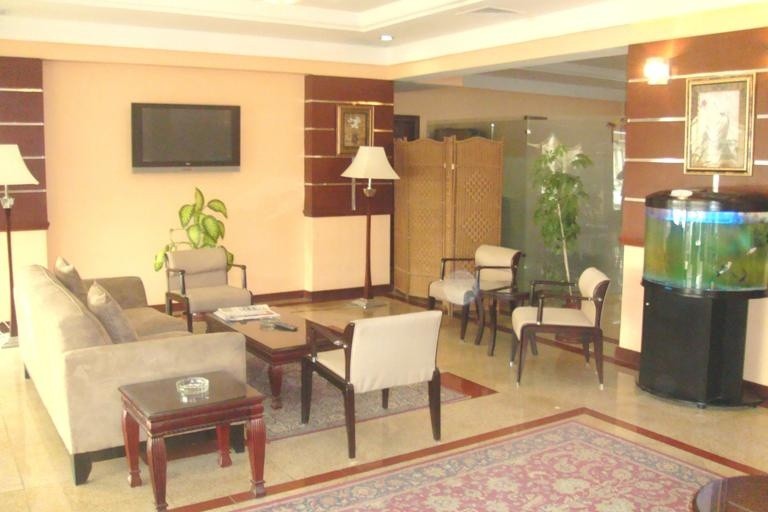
[213,304,280,322]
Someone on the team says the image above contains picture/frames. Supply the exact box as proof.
[678,69,763,179]
[333,101,377,160]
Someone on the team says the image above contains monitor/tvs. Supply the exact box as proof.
[131,103,241,167]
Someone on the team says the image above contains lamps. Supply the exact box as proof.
[339,142,403,311]
[0,142,47,348]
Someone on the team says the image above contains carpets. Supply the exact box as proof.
[155,405,766,512]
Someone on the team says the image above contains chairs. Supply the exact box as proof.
[507,265,613,395]
[297,307,449,459]
[421,239,529,349]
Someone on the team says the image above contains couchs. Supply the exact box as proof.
[5,260,256,491]
[158,246,256,334]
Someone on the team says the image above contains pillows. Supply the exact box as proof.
[84,279,143,347]
[52,254,91,297]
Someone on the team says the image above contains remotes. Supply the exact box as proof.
[274,322,297,331]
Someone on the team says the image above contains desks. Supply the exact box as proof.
[473,289,546,358]
[114,369,277,512]
